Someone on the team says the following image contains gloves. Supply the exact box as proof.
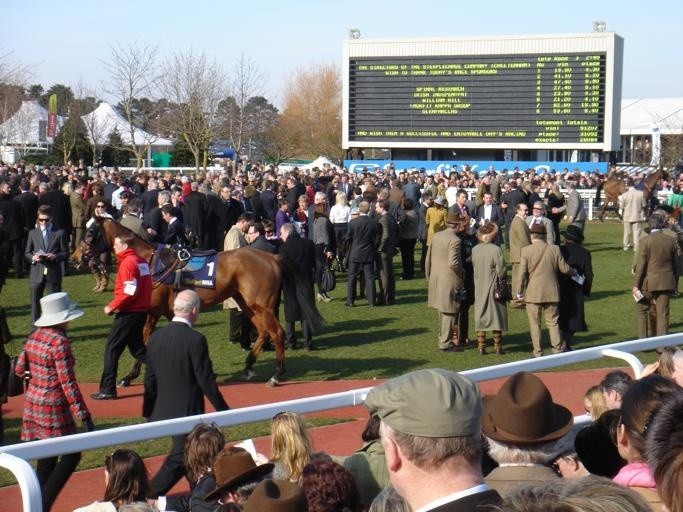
[82,416,93,432]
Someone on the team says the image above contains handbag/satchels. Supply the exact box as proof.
[7,357,23,396]
[319,269,334,291]
[494,284,511,299]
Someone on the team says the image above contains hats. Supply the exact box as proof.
[202,446,308,511]
[33,291,83,327]
[529,224,545,234]
[364,368,627,478]
[433,197,443,205]
[446,212,460,223]
[477,223,498,241]
[561,225,583,242]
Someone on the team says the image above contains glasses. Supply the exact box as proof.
[38,219,48,222]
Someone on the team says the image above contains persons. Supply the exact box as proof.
[140,288,232,502]
[223,212,320,350]
[344,344,682,512]
[90,231,152,399]
[2,156,682,308]
[78,410,358,512]
[632,209,679,339]
[14,291,94,511]
[424,212,593,358]
[25,205,68,325]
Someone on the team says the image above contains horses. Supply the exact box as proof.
[595,166,671,223]
[69,211,327,389]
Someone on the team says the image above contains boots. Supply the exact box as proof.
[335,255,344,272]
[91,270,108,293]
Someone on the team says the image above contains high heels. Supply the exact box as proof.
[317,292,332,303]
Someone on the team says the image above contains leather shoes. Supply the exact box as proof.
[91,393,115,400]
[250,342,318,351]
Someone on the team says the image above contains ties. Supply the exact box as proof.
[43,230,48,253]
